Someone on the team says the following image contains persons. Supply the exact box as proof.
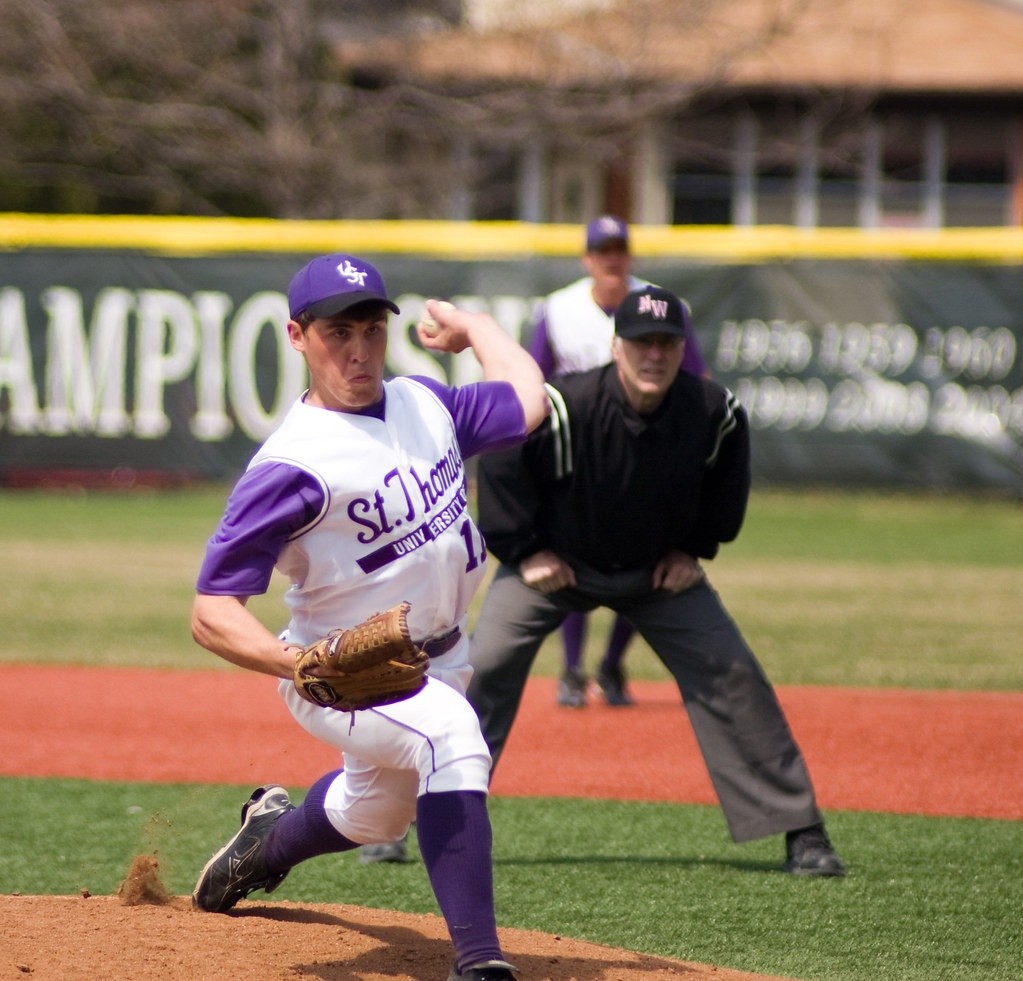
[525,212,709,711]
[363,283,848,873]
[189,253,550,981]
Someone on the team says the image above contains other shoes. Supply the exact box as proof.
[560,672,588,707]
[596,667,627,704]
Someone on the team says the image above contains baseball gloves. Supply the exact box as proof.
[281,599,434,738]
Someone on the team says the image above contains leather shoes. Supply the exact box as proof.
[356,832,406,863]
[782,823,845,876]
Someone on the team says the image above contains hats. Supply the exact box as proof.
[288,252,400,324]
[615,286,690,340]
[586,214,627,246]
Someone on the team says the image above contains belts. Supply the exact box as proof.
[414,624,461,658]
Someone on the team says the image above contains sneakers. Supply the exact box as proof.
[446,960,521,981]
[193,783,295,913]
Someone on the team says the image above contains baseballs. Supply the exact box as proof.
[421,299,459,338]
